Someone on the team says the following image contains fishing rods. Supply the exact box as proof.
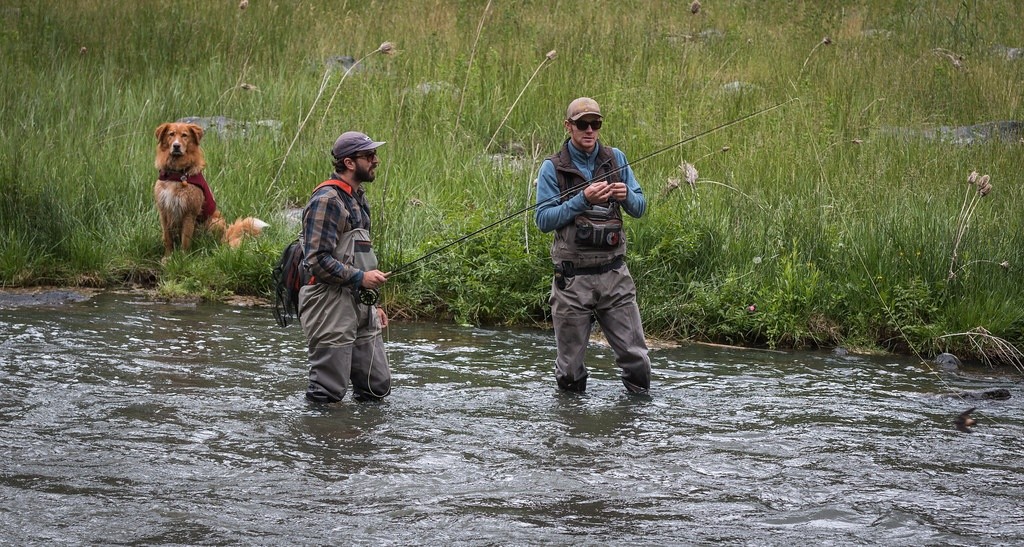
[384,98,802,279]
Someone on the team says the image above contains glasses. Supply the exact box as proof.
[350,149,377,161]
[569,120,602,130]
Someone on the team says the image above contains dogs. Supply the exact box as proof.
[154,122,273,264]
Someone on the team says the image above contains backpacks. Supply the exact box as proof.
[272,184,372,319]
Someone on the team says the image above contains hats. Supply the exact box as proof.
[331,132,386,159]
[566,98,603,120]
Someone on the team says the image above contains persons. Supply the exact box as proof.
[298,131,391,402]
[535,96,651,397]
